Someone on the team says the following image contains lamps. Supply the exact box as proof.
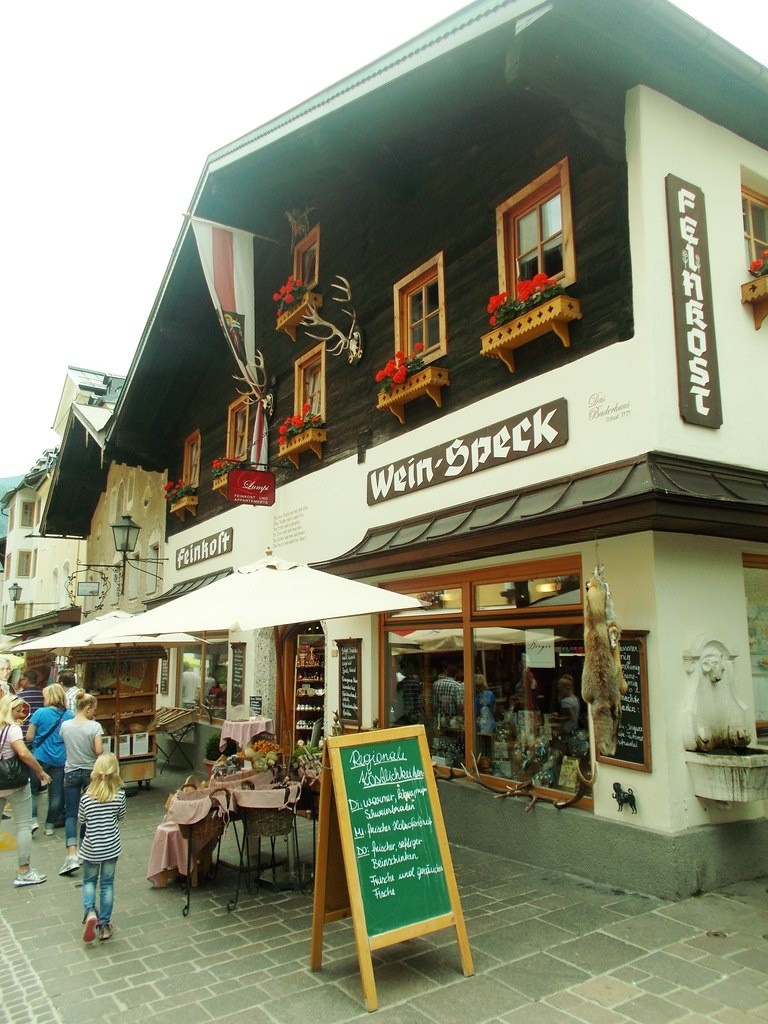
[110,513,171,580]
[536,582,560,594]
[8,583,59,607]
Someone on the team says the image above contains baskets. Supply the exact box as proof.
[244,807,291,835]
[172,784,230,840]
[155,707,198,733]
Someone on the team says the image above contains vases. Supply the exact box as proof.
[170,491,198,514]
[293,223,307,237]
[275,289,323,334]
[212,473,229,493]
[376,364,449,410]
[202,704,228,724]
[480,289,581,358]
[278,418,325,460]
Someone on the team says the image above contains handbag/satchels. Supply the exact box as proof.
[0,724,28,790]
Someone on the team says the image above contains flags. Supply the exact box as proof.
[191,217,268,473]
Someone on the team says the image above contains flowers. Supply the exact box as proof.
[277,403,322,442]
[163,479,199,504]
[211,456,245,477]
[749,248,768,276]
[206,684,230,707]
[489,274,557,322]
[289,206,310,227]
[375,342,427,393]
[272,278,304,319]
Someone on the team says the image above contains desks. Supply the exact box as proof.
[220,716,272,754]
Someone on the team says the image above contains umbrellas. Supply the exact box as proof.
[388,609,565,655]
[11,607,214,760]
[86,549,432,774]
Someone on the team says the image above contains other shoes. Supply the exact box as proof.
[54,821,65,828]
[58,858,79,876]
[98,922,114,940]
[13,870,47,885]
[2,812,11,820]
[31,822,39,835]
[80,859,83,865]
[83,912,97,942]
[43,824,54,835]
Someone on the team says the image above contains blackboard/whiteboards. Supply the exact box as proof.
[595,629,653,773]
[335,638,362,725]
[160,648,170,695]
[230,642,246,706]
[313,724,463,952]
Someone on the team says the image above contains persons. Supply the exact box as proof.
[60,689,104,876]
[0,658,17,700]
[26,684,75,838]
[548,674,579,731]
[16,670,45,747]
[396,662,496,734]
[56,670,81,717]
[0,693,52,885]
[77,753,126,942]
[181,661,216,706]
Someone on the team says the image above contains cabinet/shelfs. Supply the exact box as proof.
[294,665,323,733]
[80,656,160,763]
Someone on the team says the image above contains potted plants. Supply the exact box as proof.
[205,732,221,778]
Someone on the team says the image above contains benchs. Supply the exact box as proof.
[740,274,768,303]
